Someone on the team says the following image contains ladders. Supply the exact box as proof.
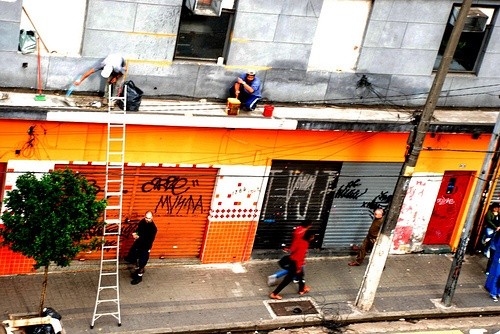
[90,83,127,329]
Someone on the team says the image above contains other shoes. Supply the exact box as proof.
[130,276,142,285]
[348,261,361,266]
[489,294,498,302]
[298,285,310,295]
[293,279,306,284]
[269,292,283,300]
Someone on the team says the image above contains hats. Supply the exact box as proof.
[101,64,113,78]
[246,69,256,75]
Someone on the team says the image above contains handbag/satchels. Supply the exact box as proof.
[278,255,297,274]
[117,80,144,112]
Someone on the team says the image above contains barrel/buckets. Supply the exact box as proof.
[225,98,241,116]
[263,105,274,117]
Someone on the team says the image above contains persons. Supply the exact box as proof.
[73,52,128,109]
[267,219,314,286]
[229,71,261,112]
[269,227,316,298]
[126,211,158,285]
[477,202,500,300]
[348,209,386,271]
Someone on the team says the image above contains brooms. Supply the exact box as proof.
[35,37,47,100]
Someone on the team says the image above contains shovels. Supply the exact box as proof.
[35,37,46,100]
